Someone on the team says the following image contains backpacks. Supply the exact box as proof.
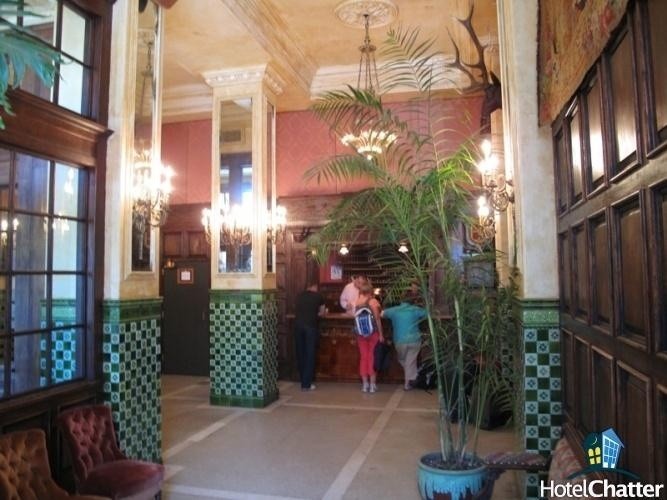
[410,359,438,389]
[351,298,379,337]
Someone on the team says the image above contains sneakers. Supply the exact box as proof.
[359,383,378,394]
[302,383,318,391]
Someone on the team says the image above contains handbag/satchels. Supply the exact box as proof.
[372,340,392,375]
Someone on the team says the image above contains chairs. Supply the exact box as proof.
[0,395,170,500]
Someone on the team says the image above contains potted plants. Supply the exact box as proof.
[304,21,524,499]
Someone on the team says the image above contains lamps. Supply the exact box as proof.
[339,12,398,163]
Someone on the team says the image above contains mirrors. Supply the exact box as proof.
[213,91,257,280]
[264,98,279,279]
[125,0,163,279]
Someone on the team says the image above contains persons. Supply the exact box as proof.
[294,281,326,392]
[381,291,430,391]
[340,275,374,314]
[350,284,387,393]
